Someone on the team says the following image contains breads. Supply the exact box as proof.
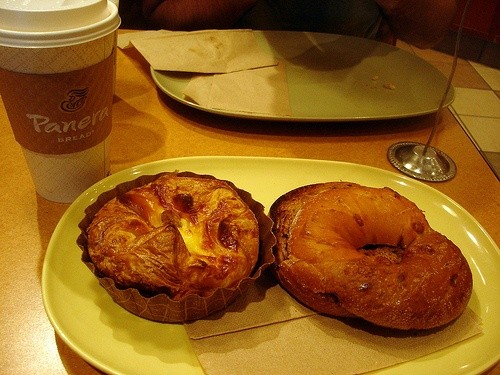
[85,176,259,300]
[268,181,474,330]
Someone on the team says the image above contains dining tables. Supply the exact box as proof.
[0,29,500,375]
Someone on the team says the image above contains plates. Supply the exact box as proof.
[150,31,456,122]
[41,156,500,375]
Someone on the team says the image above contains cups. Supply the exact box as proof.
[0,0,123,203]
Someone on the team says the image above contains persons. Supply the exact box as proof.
[118,0,457,50]
